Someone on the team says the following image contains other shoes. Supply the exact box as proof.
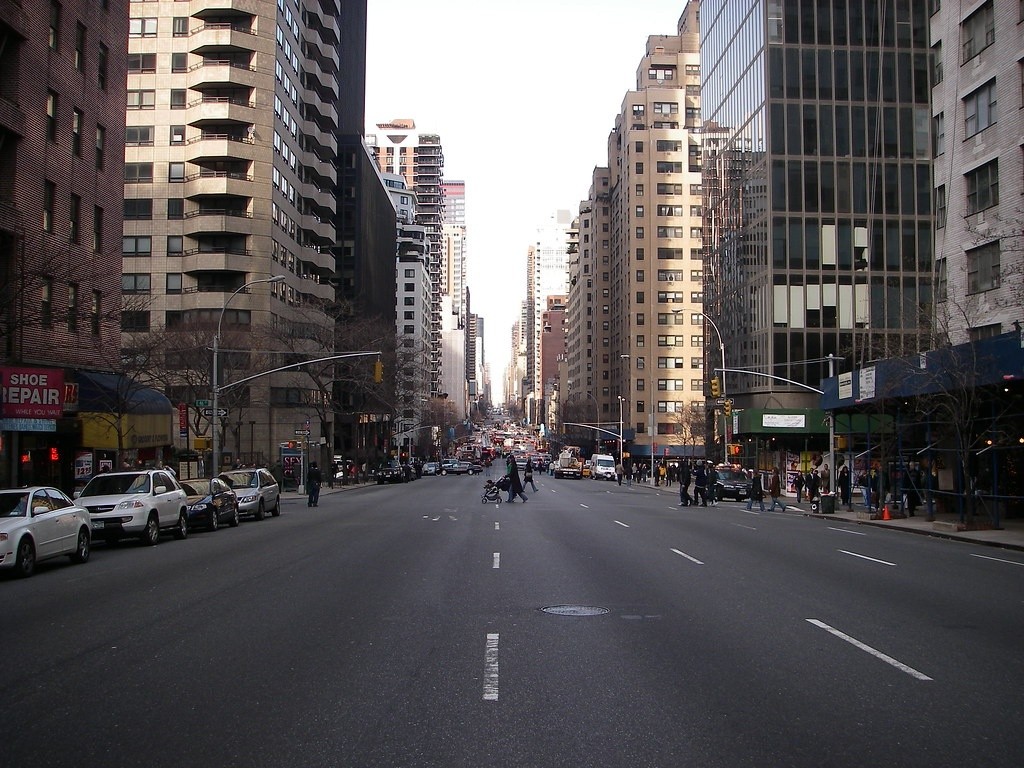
[523,498,528,503]
[505,500,514,503]
[679,502,687,506]
[308,503,312,507]
[689,499,693,505]
[783,506,786,511]
[534,489,538,492]
[313,504,319,507]
[768,509,774,511]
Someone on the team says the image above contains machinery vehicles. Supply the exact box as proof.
[553,444,585,480]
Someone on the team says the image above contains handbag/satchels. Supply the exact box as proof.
[526,472,531,477]
[502,478,510,491]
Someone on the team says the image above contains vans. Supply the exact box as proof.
[588,454,616,481]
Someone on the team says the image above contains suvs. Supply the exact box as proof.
[71,466,190,547]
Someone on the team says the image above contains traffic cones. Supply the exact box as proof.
[881,504,892,520]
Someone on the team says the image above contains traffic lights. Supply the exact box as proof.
[710,377,721,397]
[723,401,731,417]
[400,452,407,458]
[733,445,744,455]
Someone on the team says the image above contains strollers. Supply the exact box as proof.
[481,475,507,504]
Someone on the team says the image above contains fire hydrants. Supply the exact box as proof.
[810,496,820,513]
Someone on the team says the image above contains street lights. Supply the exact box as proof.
[671,308,729,463]
[620,354,655,486]
[577,391,600,454]
[211,274,287,473]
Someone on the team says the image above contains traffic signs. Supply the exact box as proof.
[204,407,229,417]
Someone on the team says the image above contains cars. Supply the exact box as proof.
[0,485,92,579]
[421,443,553,477]
[175,476,241,532]
[701,465,753,502]
[216,467,281,521]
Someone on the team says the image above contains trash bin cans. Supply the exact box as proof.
[821,495,835,514]
[811,501,820,513]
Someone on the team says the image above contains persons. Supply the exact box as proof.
[903,462,922,517]
[522,461,539,492]
[857,467,872,512]
[307,462,323,507]
[101,464,113,473]
[744,467,821,513]
[333,460,340,472]
[538,462,543,475]
[677,461,717,507]
[445,452,466,461]
[501,455,528,503]
[163,465,176,476]
[821,464,830,493]
[484,457,491,477]
[262,464,268,467]
[403,460,422,483]
[232,458,256,469]
[873,466,892,511]
[549,461,555,476]
[325,460,335,489]
[120,462,133,472]
[616,461,673,487]
[545,462,549,473]
[838,465,853,506]
